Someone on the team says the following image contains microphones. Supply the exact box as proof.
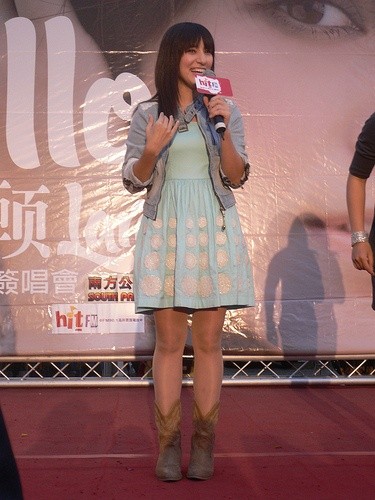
[200,69,227,134]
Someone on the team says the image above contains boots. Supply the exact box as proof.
[154,399,183,480]
[187,400,221,478]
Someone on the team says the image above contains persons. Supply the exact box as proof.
[121,21,256,480]
[0,408,24,500]
[346,112,375,310]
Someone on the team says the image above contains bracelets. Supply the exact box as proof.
[349,231,369,247]
